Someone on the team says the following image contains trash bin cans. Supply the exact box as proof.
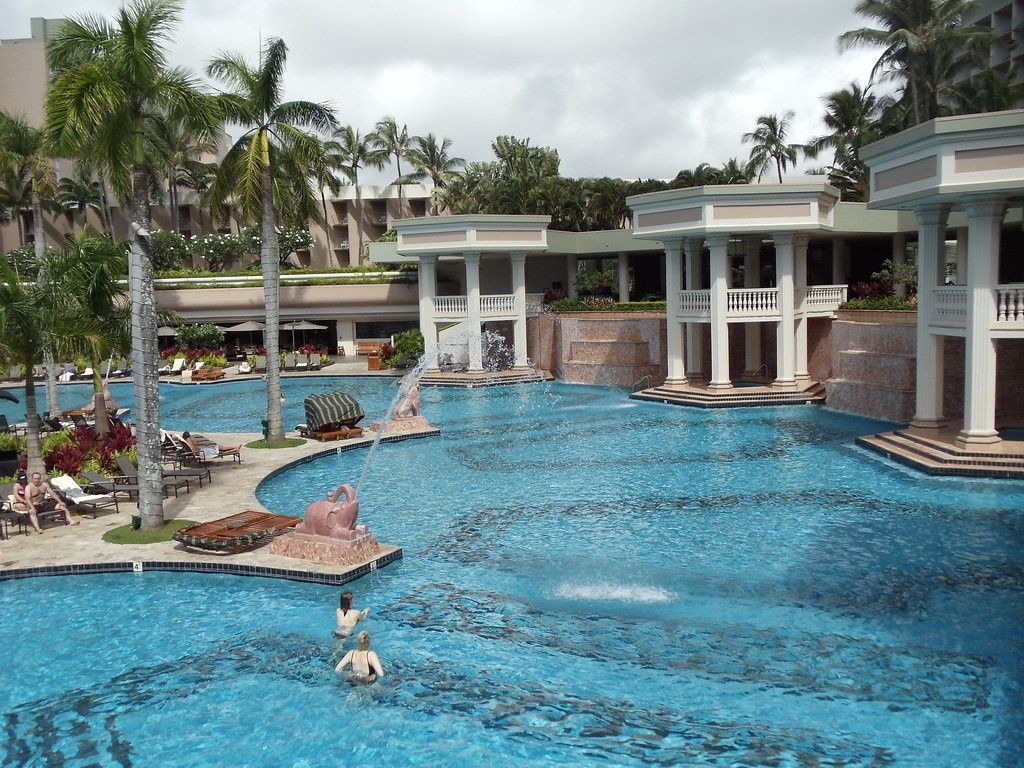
[367,350,380,371]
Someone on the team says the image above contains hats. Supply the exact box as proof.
[18,472,28,483]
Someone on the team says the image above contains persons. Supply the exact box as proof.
[30,363,81,378]
[187,360,196,371]
[325,591,370,665]
[183,431,242,456]
[334,630,385,685]
[25,472,80,534]
[12,470,30,511]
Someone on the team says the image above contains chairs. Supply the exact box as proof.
[161,467,212,488]
[160,428,186,453]
[6,493,68,536]
[339,346,345,356]
[232,348,244,361]
[296,354,308,371]
[191,367,209,381]
[108,416,126,431]
[0,496,22,540]
[70,414,95,432]
[0,415,17,437]
[51,476,120,519]
[255,356,266,373]
[309,354,321,370]
[112,362,126,379]
[284,355,295,372]
[174,433,241,465]
[206,366,222,381]
[43,419,64,431]
[182,361,204,380]
[238,362,252,373]
[59,362,75,381]
[81,367,94,379]
[23,413,54,439]
[169,359,187,374]
[80,470,140,509]
[116,455,189,498]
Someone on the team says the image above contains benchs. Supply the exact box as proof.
[356,342,380,356]
